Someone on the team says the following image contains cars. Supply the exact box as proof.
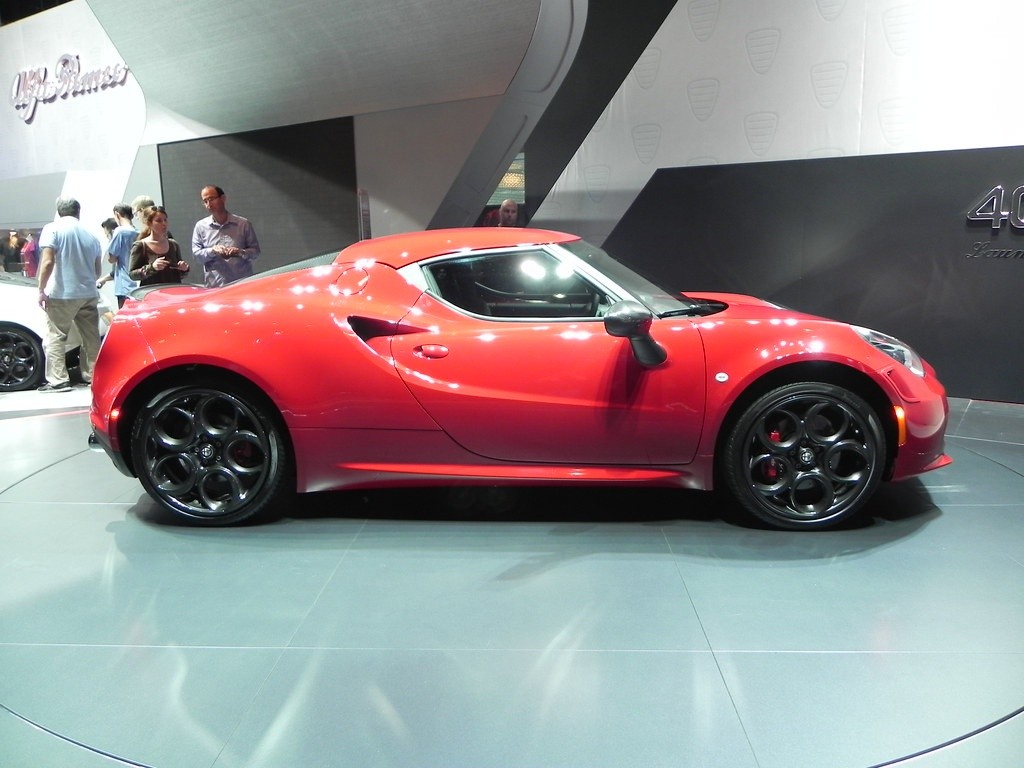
[0,270,113,392]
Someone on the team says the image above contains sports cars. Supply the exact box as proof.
[87,225,953,533]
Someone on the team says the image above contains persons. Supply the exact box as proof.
[493,200,518,227]
[0,232,38,277]
[38,199,103,392]
[97,196,190,310]
[191,185,261,287]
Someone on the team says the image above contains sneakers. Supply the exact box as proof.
[79,380,92,387]
[37,381,72,393]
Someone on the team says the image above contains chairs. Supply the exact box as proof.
[437,265,490,316]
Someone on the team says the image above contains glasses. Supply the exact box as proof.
[133,208,144,218]
[201,195,221,205]
